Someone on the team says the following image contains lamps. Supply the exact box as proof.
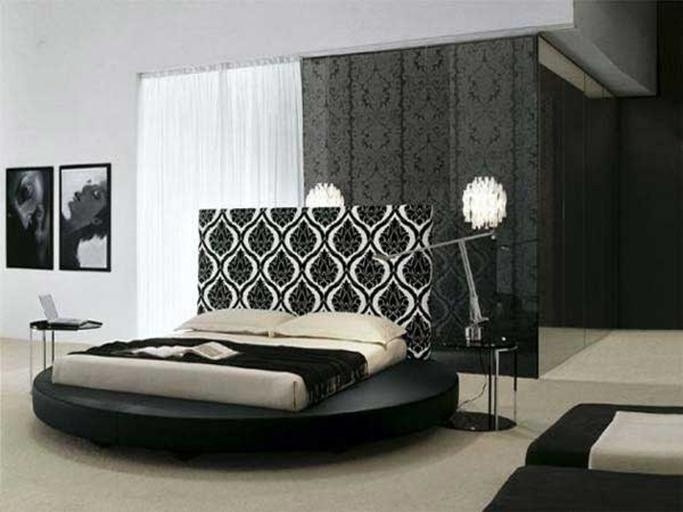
[461,176,511,252]
[305,181,345,207]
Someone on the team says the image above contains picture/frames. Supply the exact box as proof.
[5,166,54,269]
[58,163,112,271]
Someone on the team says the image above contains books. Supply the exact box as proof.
[112,341,238,360]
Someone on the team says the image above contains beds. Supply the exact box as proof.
[32,331,459,455]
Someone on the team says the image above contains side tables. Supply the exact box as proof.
[28,321,103,394]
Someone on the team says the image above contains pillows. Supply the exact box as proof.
[174,308,297,336]
[273,312,408,345]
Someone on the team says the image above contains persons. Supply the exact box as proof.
[6,170,47,266]
[61,178,108,266]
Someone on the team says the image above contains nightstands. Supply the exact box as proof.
[437,344,520,433]
